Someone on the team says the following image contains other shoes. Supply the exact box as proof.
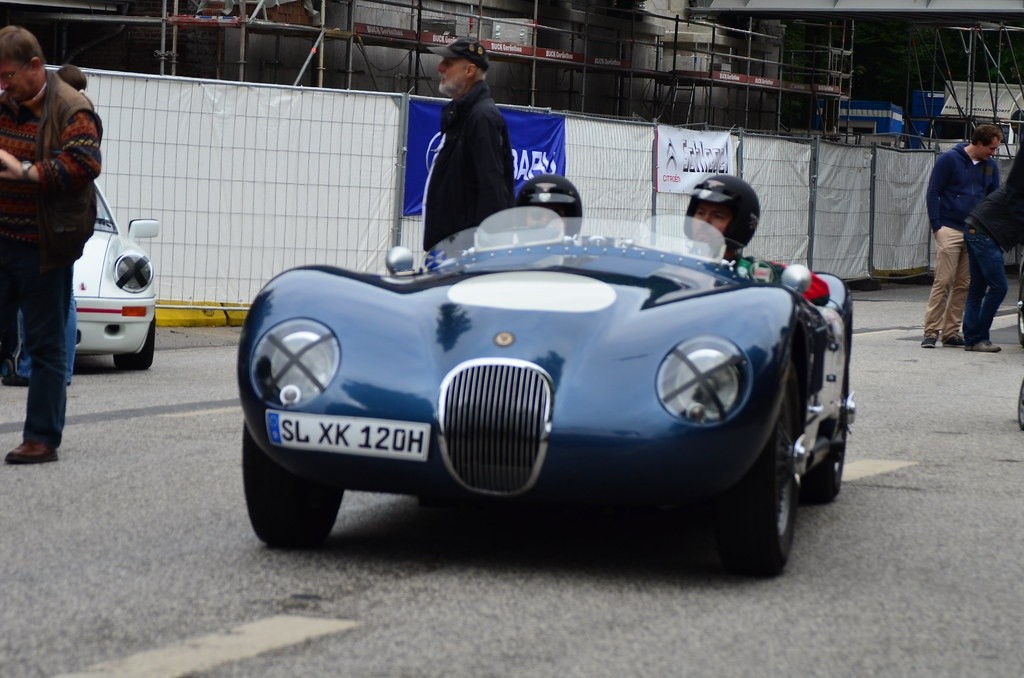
[921,335,937,348]
[943,337,967,348]
[2,375,32,387]
[4,439,58,464]
[964,340,1002,353]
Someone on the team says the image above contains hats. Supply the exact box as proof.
[425,37,489,71]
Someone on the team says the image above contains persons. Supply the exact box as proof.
[921,125,1000,348]
[964,140,1024,352]
[419,38,515,253]
[515,175,581,239]
[684,175,761,262]
[0,25,104,465]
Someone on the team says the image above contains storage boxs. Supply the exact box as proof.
[201,0,313,26]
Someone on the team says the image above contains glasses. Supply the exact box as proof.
[0,59,29,82]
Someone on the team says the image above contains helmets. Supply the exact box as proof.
[515,175,582,238]
[683,174,760,250]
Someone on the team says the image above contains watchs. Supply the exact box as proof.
[22,161,32,179]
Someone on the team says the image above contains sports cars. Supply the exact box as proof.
[73,178,161,371]
[238,206,855,575]
